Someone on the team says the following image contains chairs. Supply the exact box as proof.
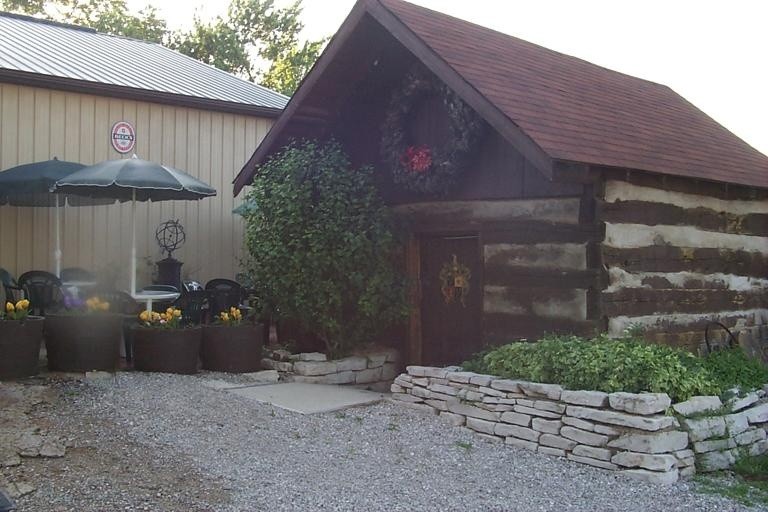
[0,267,270,347]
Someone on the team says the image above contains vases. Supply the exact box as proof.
[1,306,265,381]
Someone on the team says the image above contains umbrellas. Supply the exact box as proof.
[48,152,218,298]
[1,155,124,283]
